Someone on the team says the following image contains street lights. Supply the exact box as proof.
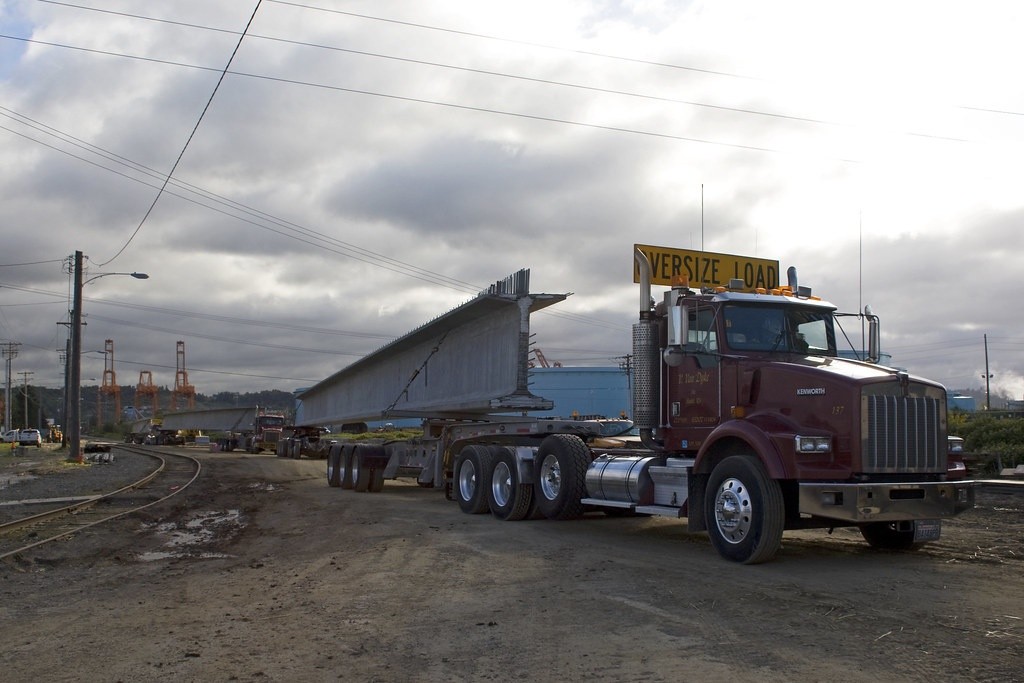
[69,273,149,462]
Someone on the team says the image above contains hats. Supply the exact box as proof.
[761,318,782,334]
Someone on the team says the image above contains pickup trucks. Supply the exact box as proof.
[0,429,21,443]
[18,429,41,448]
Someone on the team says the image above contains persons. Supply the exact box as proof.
[759,313,808,347]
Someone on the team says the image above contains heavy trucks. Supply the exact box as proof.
[296,243,975,565]
[218,413,285,454]
